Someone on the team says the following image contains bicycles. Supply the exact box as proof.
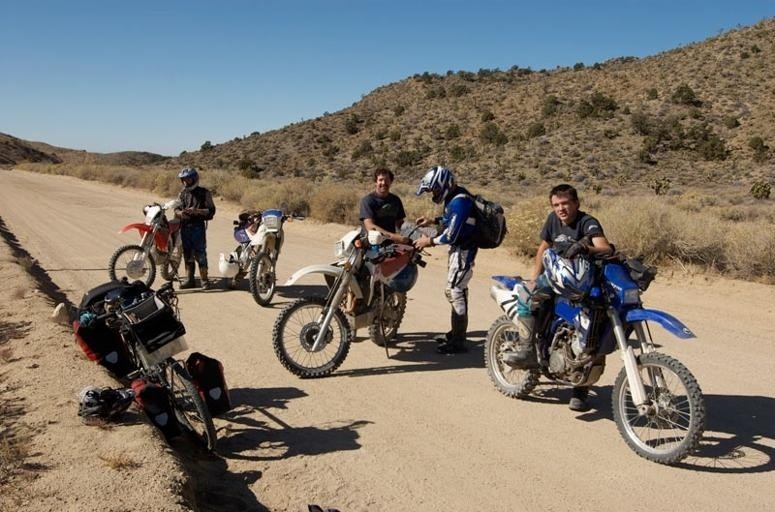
[58,274,216,459]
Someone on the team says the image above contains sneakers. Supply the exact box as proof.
[568,386,589,411]
[436,342,468,355]
[502,349,538,370]
[200,279,210,290]
[179,279,196,290]
[435,334,468,345]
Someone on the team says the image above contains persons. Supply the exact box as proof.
[347,167,411,318]
[531,184,615,412]
[175,167,216,290]
[412,166,479,354]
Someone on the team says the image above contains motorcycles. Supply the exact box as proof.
[227,208,305,307]
[106,201,184,287]
[482,231,706,466]
[271,215,432,374]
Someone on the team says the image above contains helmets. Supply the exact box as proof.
[218,252,240,279]
[541,246,597,302]
[177,166,200,192]
[415,165,455,204]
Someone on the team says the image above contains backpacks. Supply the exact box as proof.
[468,191,507,250]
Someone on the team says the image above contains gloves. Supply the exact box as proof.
[563,240,590,260]
